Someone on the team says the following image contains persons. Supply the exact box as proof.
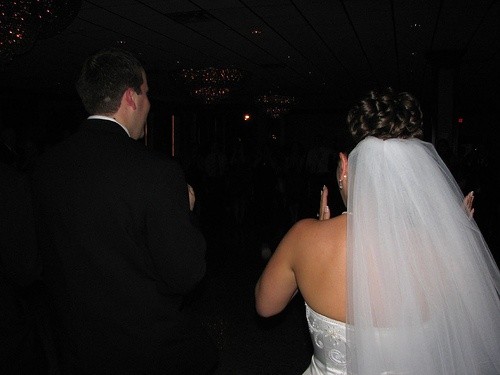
[254,87,476,375]
[24,45,221,374]
[0,163,48,374]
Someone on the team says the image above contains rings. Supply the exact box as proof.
[317,213,320,217]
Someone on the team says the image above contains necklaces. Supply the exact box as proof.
[340,210,353,216]
